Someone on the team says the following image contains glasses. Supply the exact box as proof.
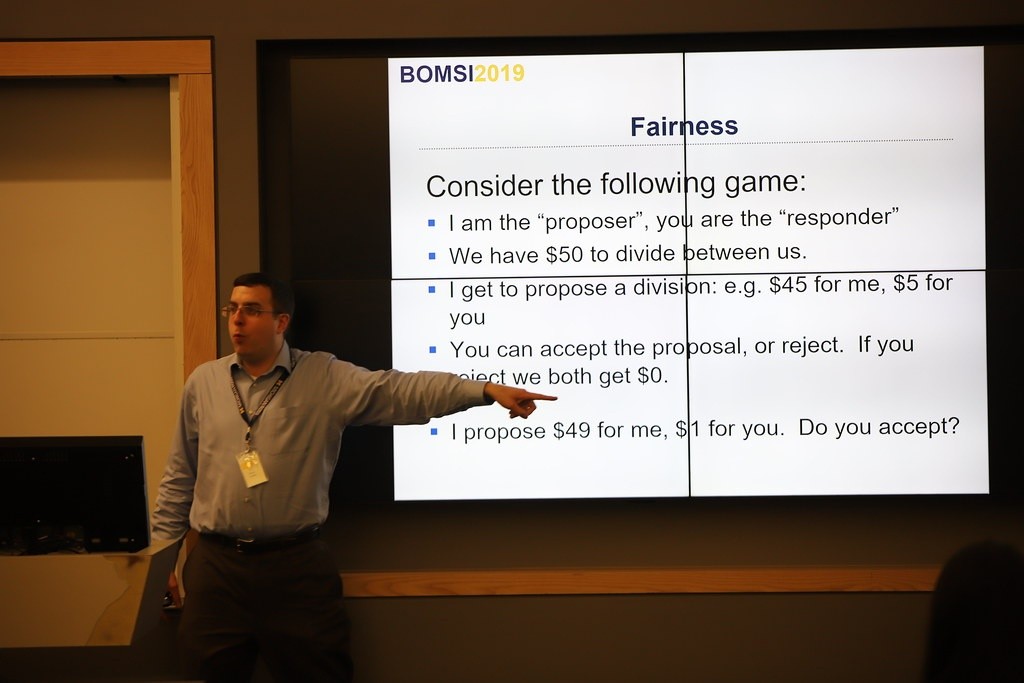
[227,303,282,318]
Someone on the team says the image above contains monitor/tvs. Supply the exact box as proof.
[0,435,151,553]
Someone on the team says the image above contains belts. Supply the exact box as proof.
[199,526,320,551]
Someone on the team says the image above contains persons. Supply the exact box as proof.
[149,273,559,682]
